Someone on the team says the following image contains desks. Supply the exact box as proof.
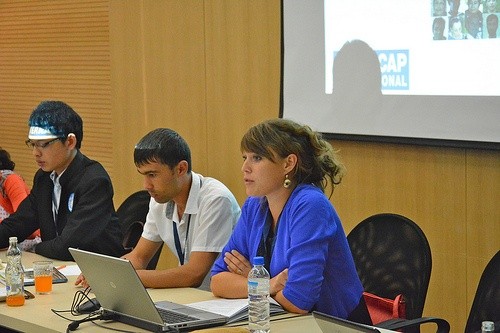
[0,249,399,333]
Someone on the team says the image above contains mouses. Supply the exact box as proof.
[77,298,101,314]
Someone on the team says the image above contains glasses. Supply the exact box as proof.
[25,137,60,151]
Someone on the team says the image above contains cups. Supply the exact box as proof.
[32,261,53,295]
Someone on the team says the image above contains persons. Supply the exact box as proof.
[486,14,498,38]
[448,17,466,39]
[465,16,482,40]
[465,0,482,14]
[0,102,124,262]
[448,0,464,16]
[484,0,500,13]
[210,118,373,326]
[0,147,42,253]
[433,0,447,16]
[432,17,447,40]
[75,127,242,288]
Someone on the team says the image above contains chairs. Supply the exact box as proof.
[116,190,164,270]
[347,213,451,333]
[465,250,500,333]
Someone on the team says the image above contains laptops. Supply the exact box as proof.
[68,247,228,333]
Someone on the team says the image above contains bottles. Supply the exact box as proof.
[481,320,495,333]
[247,257,269,333]
[5,237,25,306]
